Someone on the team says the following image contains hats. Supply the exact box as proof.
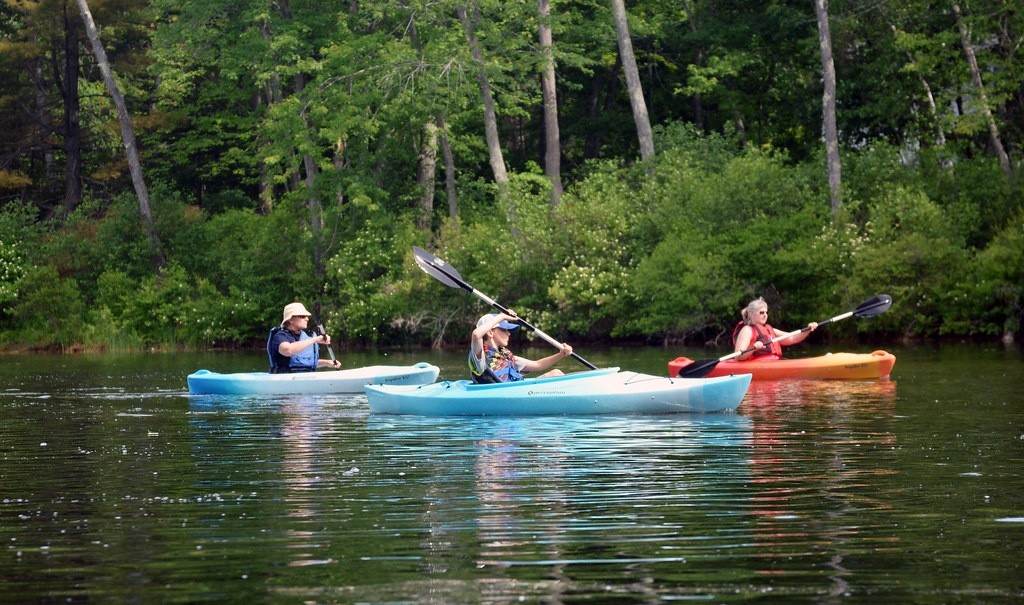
[476,313,520,332]
[282,302,312,324]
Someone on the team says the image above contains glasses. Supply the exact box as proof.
[293,315,305,319]
[754,310,769,315]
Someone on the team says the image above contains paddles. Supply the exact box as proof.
[679,293,893,378]
[411,245,598,371]
[312,299,340,371]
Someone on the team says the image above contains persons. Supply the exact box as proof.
[732,298,819,362]
[468,309,573,384]
[266,302,342,374]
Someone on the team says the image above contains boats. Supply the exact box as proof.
[363,365,753,417]
[185,362,440,396]
[666,350,896,382]
